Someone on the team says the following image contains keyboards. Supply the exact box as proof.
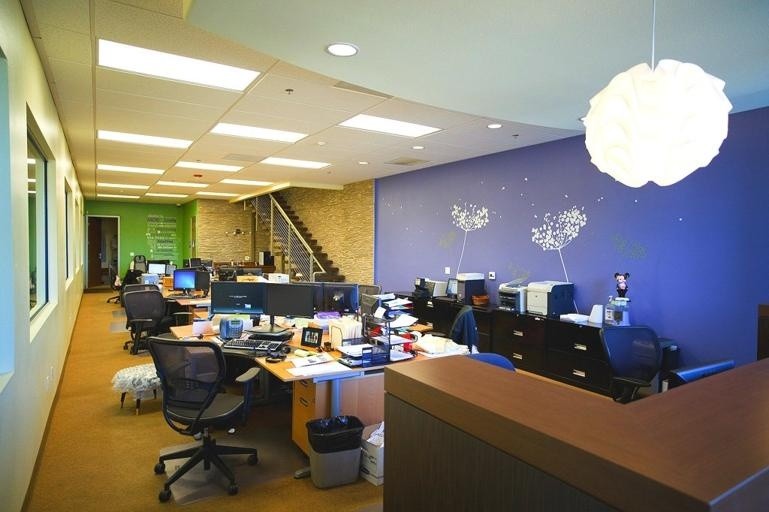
[435,296,456,302]
[168,293,193,299]
[248,333,294,341]
[224,339,282,351]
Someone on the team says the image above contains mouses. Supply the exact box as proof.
[284,346,291,353]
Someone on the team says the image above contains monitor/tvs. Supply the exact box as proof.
[195,271,209,293]
[148,263,166,277]
[219,268,237,282]
[191,258,201,267]
[201,259,212,267]
[263,283,314,334]
[183,258,189,268]
[211,281,263,333]
[173,269,197,294]
[304,282,323,314]
[446,278,458,298]
[147,260,170,271]
[324,282,359,318]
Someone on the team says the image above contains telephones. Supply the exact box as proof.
[219,319,243,339]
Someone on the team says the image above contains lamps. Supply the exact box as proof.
[577,0,733,191]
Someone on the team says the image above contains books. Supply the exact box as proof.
[327,316,362,351]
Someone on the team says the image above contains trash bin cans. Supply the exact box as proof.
[305,414,363,490]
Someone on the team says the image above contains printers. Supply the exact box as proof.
[527,281,574,317]
[492,277,527,315]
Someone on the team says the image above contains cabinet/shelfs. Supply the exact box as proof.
[393,294,633,397]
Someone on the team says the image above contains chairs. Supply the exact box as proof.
[599,324,664,402]
[121,281,260,503]
[107,255,148,304]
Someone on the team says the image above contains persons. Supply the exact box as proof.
[306,334,311,342]
[313,335,318,342]
[310,331,314,340]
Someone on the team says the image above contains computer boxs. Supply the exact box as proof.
[456,280,485,305]
